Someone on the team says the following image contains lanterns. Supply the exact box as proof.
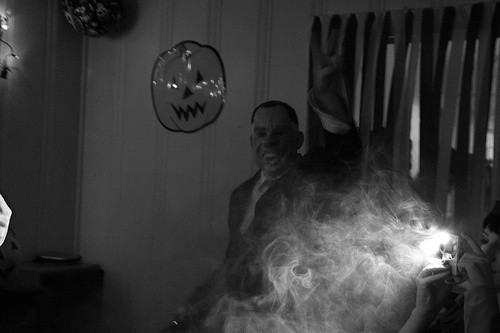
[151,41,227,134]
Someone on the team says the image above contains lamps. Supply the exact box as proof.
[0,7,21,79]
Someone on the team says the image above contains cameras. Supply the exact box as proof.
[440,232,464,276]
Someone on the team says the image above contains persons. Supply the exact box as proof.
[167,39,364,332]
[397,199,500,333]
